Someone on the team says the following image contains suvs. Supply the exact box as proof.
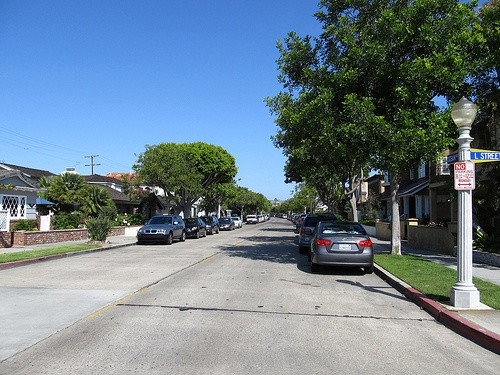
[257,215,264,222]
[201,215,220,236]
[264,214,270,220]
[298,213,337,253]
[277,213,283,218]
[296,215,306,233]
[287,215,299,225]
[230,217,243,229]
[136,216,186,244]
[245,215,259,224]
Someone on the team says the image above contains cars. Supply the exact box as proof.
[281,214,287,219]
[218,218,235,231]
[183,217,207,239]
[305,220,374,274]
